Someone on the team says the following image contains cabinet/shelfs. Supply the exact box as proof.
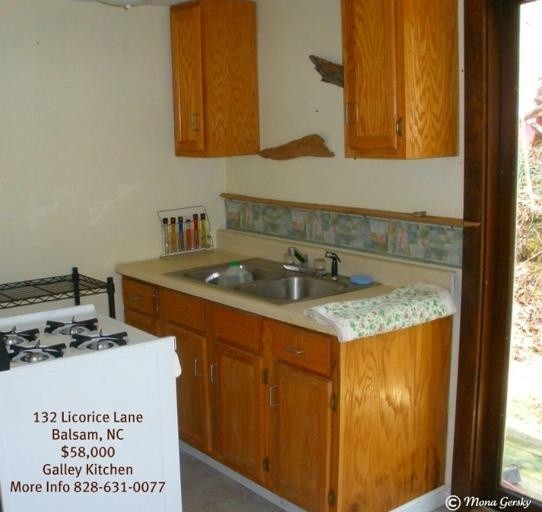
[122,274,453,512]
[171,0,260,157]
[341,0,456,160]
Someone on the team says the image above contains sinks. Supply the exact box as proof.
[172,256,290,288]
[236,269,383,304]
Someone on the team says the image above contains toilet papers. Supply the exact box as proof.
[350,274,374,286]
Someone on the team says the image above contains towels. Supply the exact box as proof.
[306,281,458,344]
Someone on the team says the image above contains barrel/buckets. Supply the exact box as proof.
[217,260,254,289]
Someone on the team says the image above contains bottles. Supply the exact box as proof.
[162,218,170,254]
[184,220,191,251]
[177,217,184,252]
[169,218,177,253]
[192,214,199,249]
[199,213,207,249]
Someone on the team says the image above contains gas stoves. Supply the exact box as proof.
[3,315,169,369]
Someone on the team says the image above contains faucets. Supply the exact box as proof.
[289,246,311,274]
[324,249,343,276]
